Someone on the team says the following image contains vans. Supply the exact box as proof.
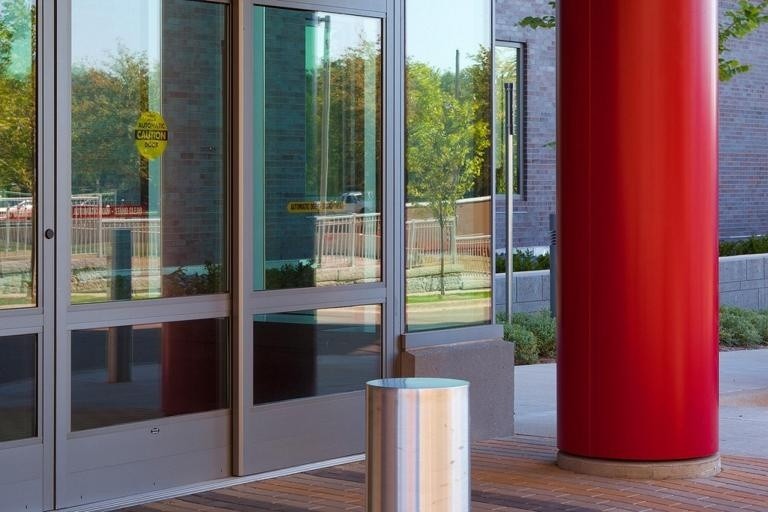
[339,190,364,212]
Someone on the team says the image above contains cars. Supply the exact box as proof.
[6,198,32,220]
